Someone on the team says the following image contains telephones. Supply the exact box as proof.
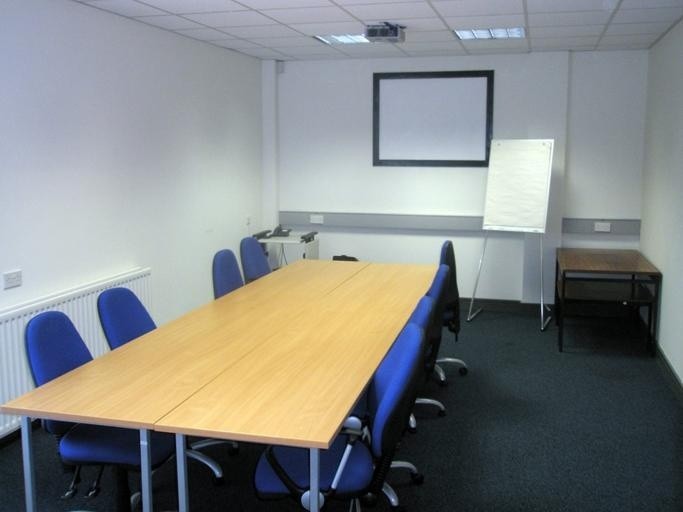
[273,224,291,236]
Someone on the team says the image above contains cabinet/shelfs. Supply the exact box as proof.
[554,246,663,357]
[258,234,318,271]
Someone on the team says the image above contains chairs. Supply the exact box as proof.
[428,262,450,349]
[241,238,269,282]
[23,309,175,508]
[349,295,447,430]
[253,320,426,512]
[95,287,241,489]
[429,239,466,388]
[211,250,244,297]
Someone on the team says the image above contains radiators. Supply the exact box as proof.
[0,265,152,439]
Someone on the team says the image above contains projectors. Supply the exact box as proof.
[364,26,405,44]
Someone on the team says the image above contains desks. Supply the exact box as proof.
[1,260,371,511]
[153,263,440,509]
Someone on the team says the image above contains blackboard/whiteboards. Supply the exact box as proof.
[482,139,555,233]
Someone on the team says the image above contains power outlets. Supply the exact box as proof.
[3,270,22,290]
